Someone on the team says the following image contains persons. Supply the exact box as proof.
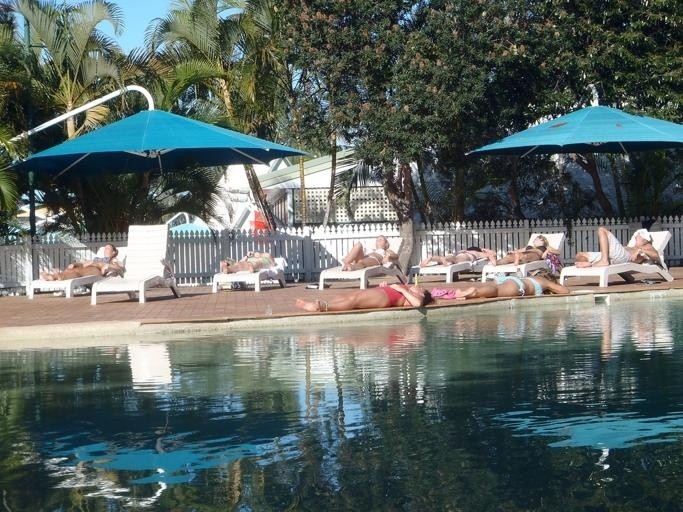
[495,304,570,339]
[295,323,430,352]
[487,233,560,266]
[419,245,498,267]
[295,280,431,310]
[341,234,399,271]
[574,226,662,269]
[222,250,276,273]
[452,269,573,300]
[39,243,124,281]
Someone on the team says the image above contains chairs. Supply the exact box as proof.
[480,230,677,290]
[28,224,181,305]
[215,250,499,289]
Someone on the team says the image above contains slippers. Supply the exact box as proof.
[430,287,457,300]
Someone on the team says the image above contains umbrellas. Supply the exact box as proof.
[0,85,320,185]
[466,85,682,159]
